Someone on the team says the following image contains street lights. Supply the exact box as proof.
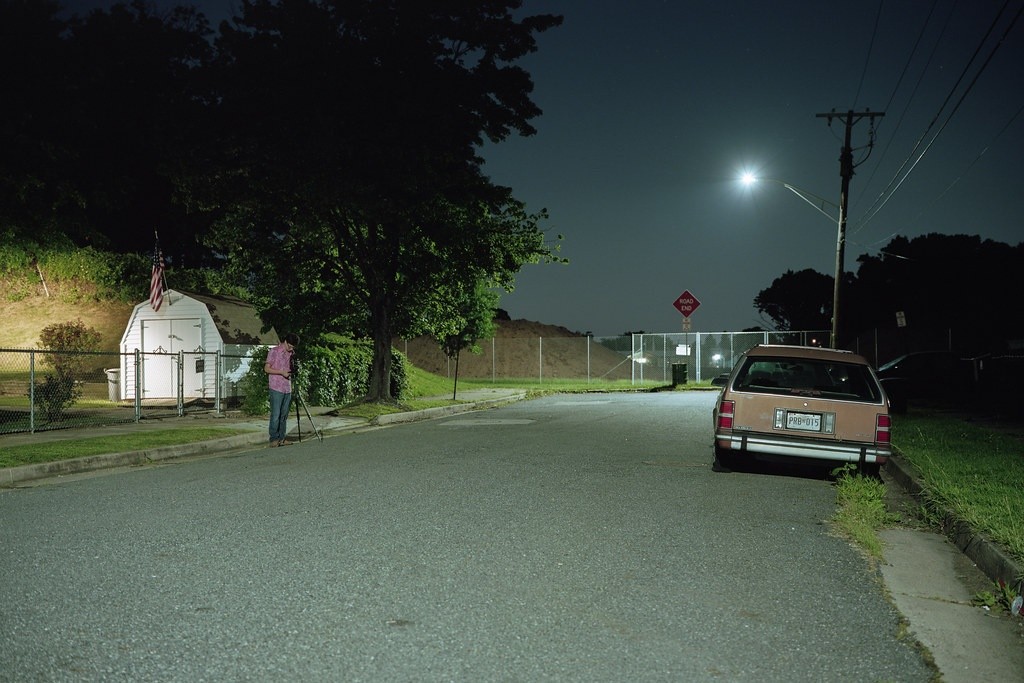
[738,174,846,347]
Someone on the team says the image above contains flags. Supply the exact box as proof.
[150,239,166,313]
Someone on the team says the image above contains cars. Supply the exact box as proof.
[710,342,892,480]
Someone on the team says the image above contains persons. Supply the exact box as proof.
[264,334,299,448]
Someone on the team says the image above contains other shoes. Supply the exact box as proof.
[269,439,294,447]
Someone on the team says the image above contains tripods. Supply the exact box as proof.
[286,378,322,443]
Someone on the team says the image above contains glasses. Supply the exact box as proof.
[287,342,296,349]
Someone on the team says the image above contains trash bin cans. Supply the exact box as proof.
[103,368,120,401]
[878,377,907,414]
